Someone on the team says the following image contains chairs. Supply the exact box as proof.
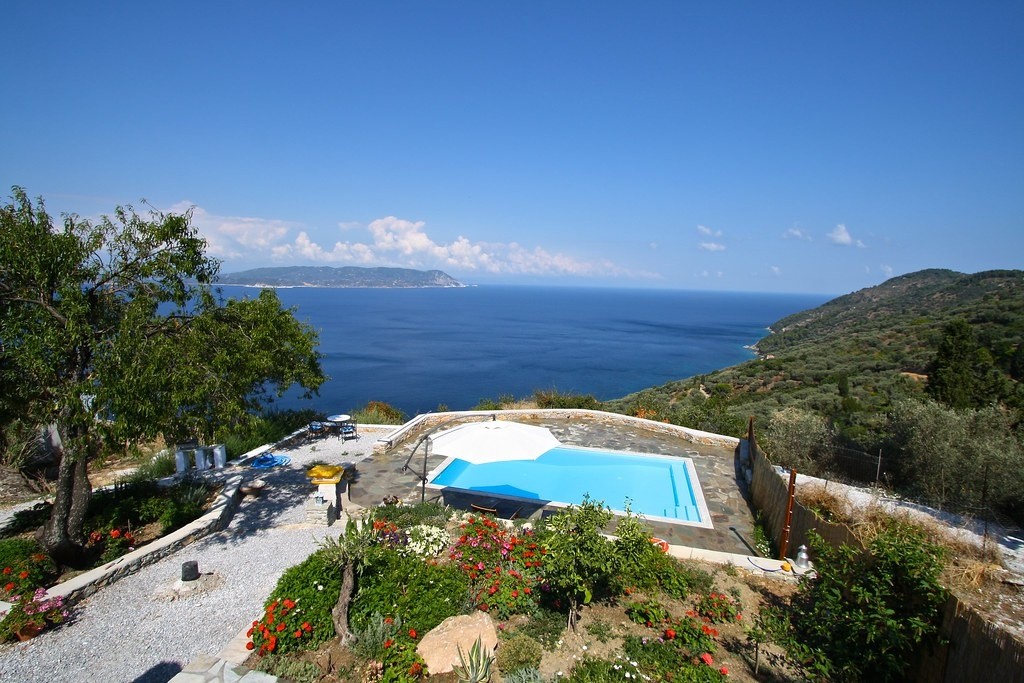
[470,502,524,520]
[338,419,357,444]
[308,420,326,439]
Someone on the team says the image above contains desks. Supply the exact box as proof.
[327,415,350,438]
